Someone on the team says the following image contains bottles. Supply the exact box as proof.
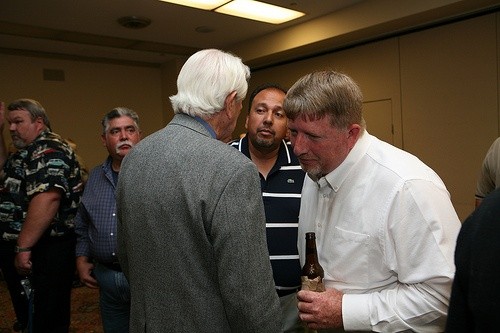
[18,268,32,299]
[301,231,325,293]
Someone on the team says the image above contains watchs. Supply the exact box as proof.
[16,246,32,253]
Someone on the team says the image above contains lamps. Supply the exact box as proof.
[117,17,152,28]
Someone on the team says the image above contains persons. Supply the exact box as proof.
[444,137,500,333]
[282,70,462,333]
[74,107,142,333]
[226,82,306,333]
[115,48,282,333]
[0,99,87,333]
